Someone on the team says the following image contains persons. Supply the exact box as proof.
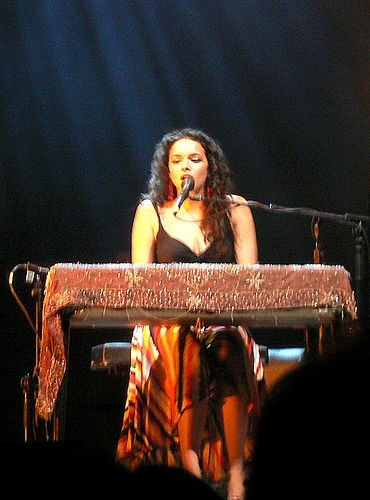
[111,125,265,500]
[0,424,225,500]
[241,321,370,500]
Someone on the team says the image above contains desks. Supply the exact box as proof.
[51,307,343,445]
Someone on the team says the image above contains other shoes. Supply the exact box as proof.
[227,476,246,500]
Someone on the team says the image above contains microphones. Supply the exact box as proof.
[26,263,50,275]
[172,176,195,216]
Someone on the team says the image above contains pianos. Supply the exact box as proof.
[49,262,351,328]
